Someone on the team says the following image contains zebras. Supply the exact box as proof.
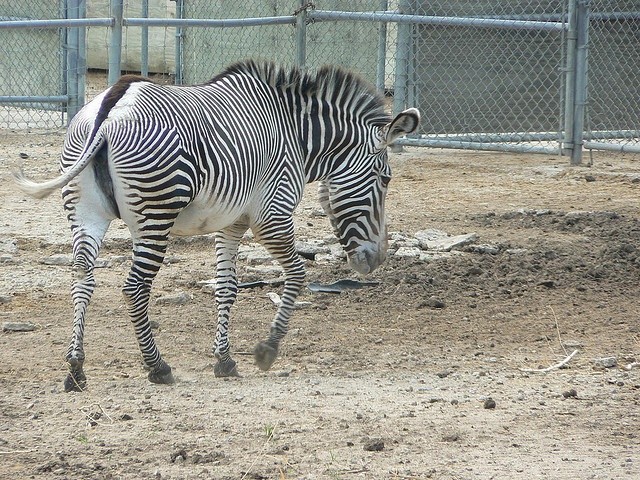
[9,57,422,394]
